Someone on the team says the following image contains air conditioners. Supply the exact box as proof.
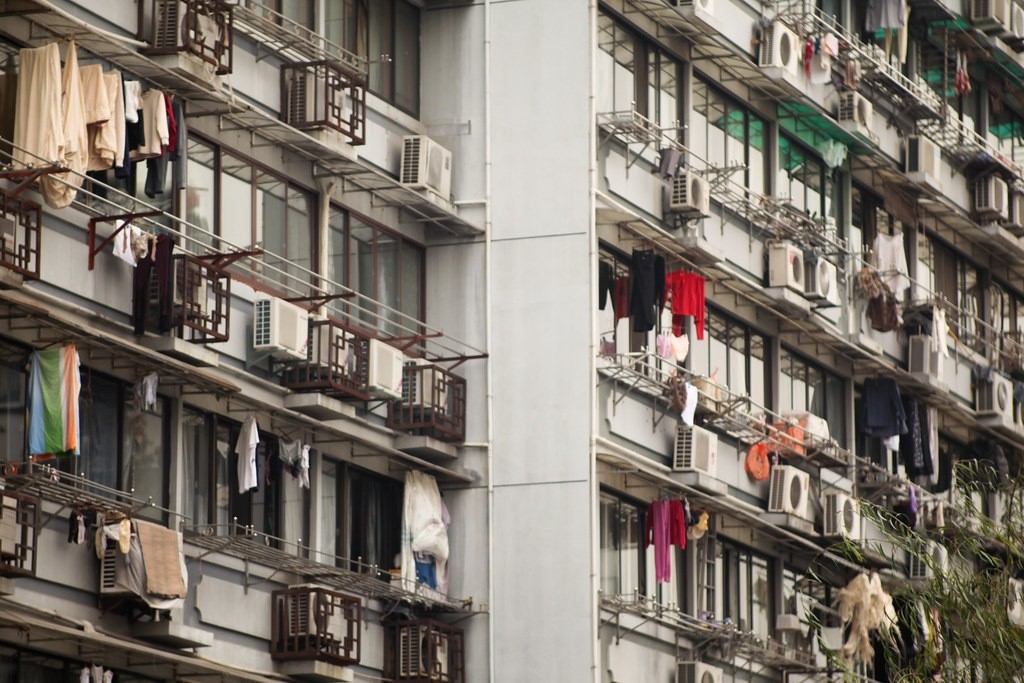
[804,257,838,306]
[354,338,402,400]
[100,518,166,601]
[297,315,362,384]
[141,0,221,70]
[663,0,1024,242]
[146,256,208,321]
[908,335,949,392]
[768,243,804,293]
[398,358,448,415]
[400,625,448,681]
[974,372,1013,421]
[253,297,308,361]
[288,583,344,644]
[401,134,452,201]
[674,423,1024,683]
[287,73,357,142]
